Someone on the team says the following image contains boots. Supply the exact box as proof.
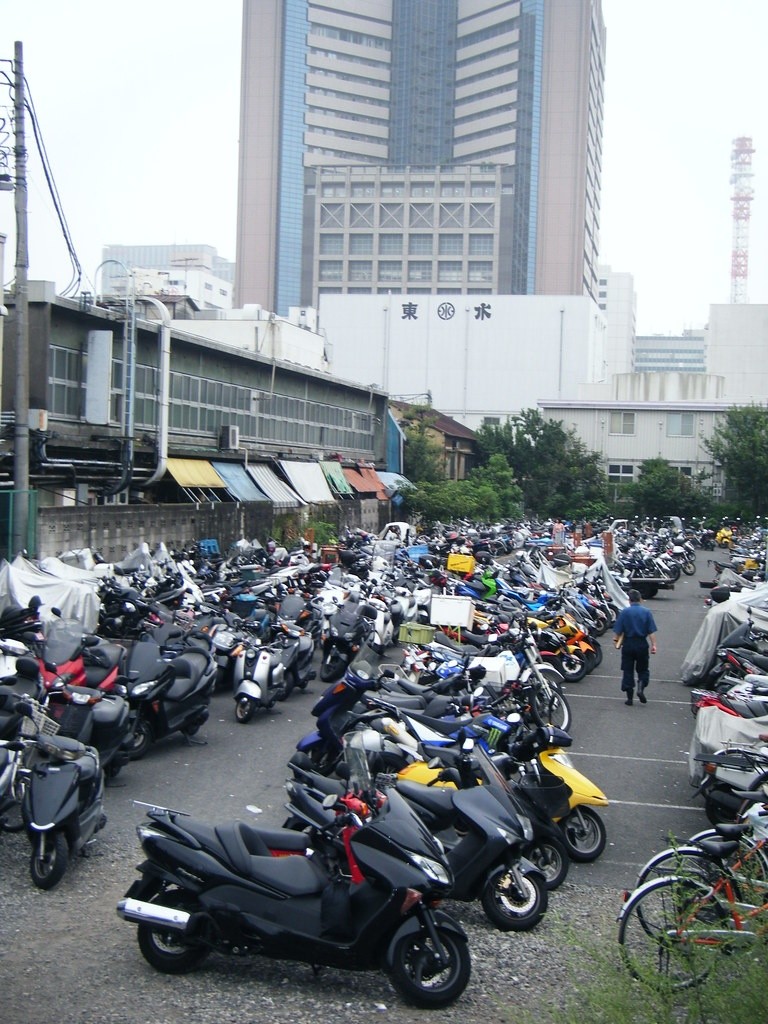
[636,681,647,703]
[625,689,634,706]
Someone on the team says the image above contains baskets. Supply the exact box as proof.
[519,773,571,818]
[21,709,62,737]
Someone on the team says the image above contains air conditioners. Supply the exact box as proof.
[220,425,240,451]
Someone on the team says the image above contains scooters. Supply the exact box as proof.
[0,514,768,1001]
[10,701,106,892]
[114,727,472,1012]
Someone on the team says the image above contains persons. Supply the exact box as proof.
[552,518,566,538]
[612,589,658,707]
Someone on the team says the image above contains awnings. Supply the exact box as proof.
[164,458,298,508]
[279,459,417,503]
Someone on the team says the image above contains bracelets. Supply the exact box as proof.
[613,640,618,642]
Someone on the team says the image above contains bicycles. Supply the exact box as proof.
[1,692,61,832]
[616,811,768,993]
[633,824,768,954]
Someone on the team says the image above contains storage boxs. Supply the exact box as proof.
[430,593,475,631]
[398,623,435,645]
[447,554,476,575]
